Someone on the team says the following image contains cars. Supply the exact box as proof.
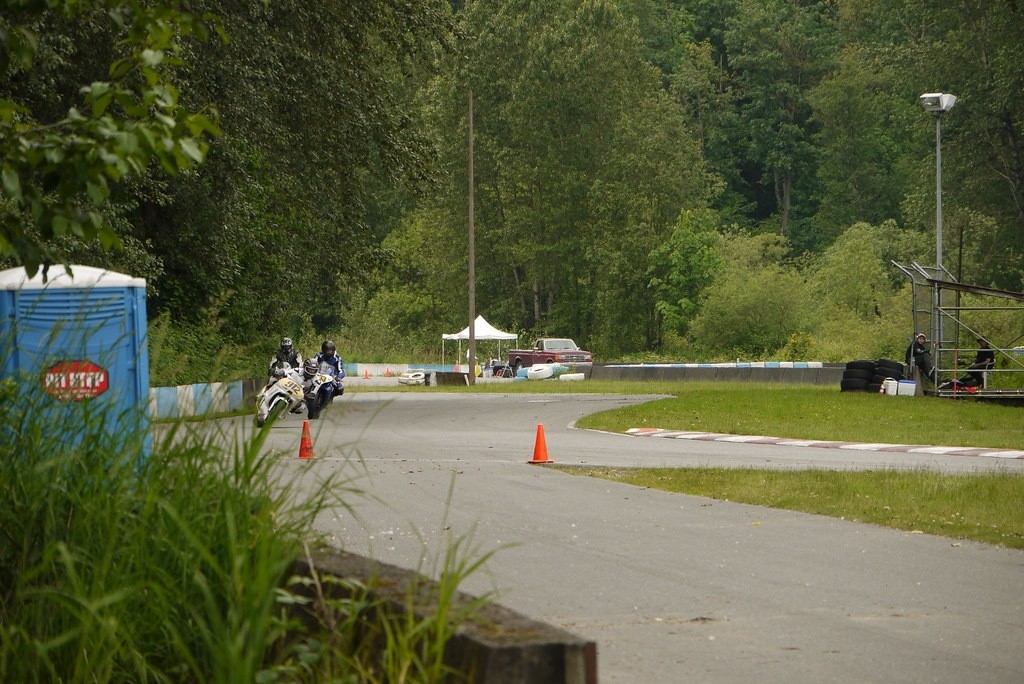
[397,368,425,385]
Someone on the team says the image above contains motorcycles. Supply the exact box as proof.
[304,365,344,420]
[252,361,305,428]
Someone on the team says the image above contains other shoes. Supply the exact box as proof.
[938,381,950,389]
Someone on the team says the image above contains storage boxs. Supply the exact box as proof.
[897,379,917,396]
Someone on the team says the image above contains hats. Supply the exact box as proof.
[976,336,989,344]
[916,334,926,341]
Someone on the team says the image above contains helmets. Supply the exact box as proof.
[281,338,292,353]
[304,359,319,379]
[322,341,335,356]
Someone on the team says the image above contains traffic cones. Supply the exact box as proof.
[385,367,390,377]
[527,424,554,464]
[295,421,319,459]
[363,370,370,379]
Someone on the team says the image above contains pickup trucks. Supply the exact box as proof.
[507,338,592,376]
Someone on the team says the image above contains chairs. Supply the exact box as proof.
[499,361,513,377]
[971,357,996,389]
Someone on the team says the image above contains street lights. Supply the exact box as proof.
[918,92,959,379]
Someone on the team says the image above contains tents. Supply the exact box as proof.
[441,314,519,366]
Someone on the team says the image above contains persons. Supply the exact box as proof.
[268,337,305,371]
[267,358,318,415]
[967,336,994,389]
[314,340,345,411]
[905,333,950,389]
